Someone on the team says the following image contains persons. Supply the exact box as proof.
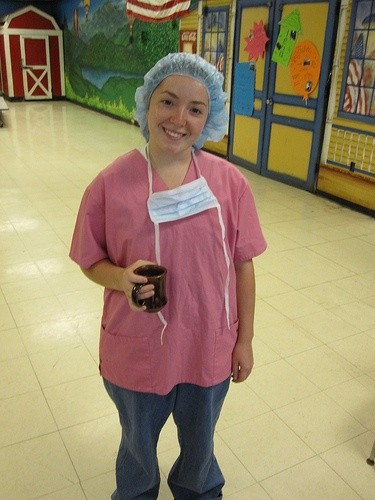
[68,52,271,500]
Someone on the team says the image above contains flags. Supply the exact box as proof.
[126,0,199,23]
[341,34,368,114]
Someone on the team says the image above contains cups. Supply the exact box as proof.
[132,264,168,313]
